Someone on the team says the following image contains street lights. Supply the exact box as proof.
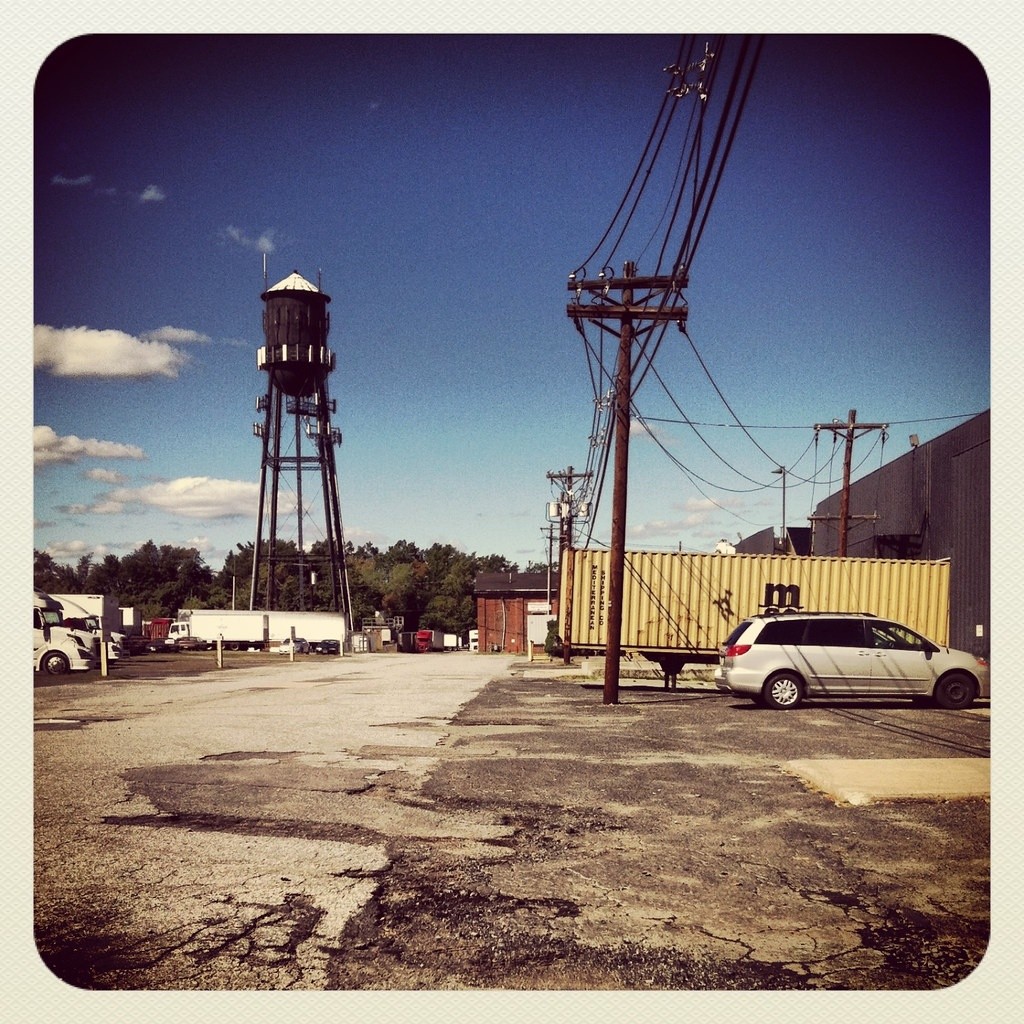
[771,465,786,555]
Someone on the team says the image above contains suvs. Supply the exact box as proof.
[714,612,990,712]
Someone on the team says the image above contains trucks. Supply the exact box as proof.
[116,607,176,655]
[34,586,96,675]
[414,630,444,653]
[443,633,463,651]
[42,594,131,666]
[469,629,479,651]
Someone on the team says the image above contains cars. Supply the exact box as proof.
[279,637,310,655]
[175,636,208,653]
[315,639,340,655]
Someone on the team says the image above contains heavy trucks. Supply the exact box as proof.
[165,609,345,651]
